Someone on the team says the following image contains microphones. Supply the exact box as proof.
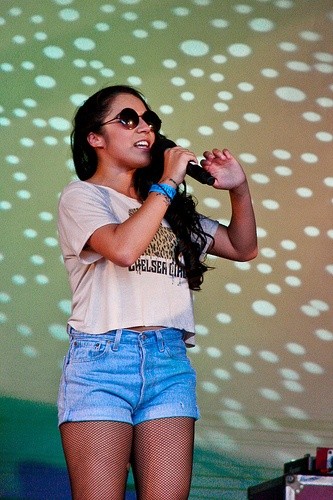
[149,138,215,185]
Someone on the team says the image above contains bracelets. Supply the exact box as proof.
[148,183,176,200]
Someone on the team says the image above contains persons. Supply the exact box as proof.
[57,85,258,500]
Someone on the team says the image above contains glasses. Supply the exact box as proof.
[93,108,162,129]
